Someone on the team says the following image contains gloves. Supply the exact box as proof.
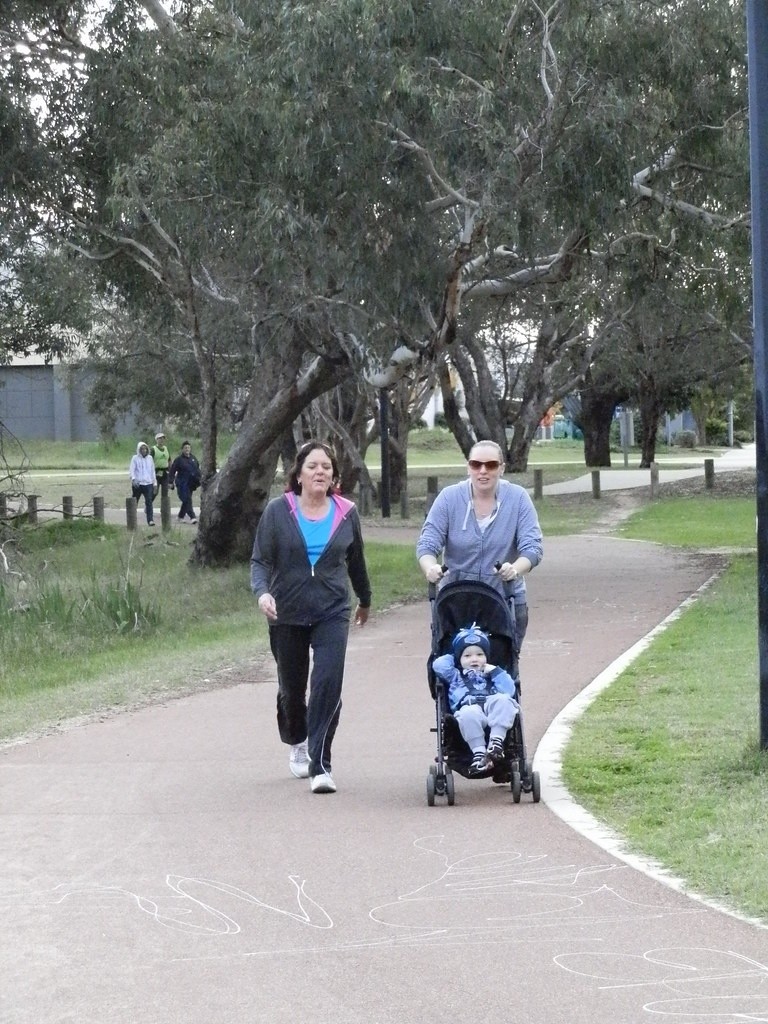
[167,484,174,489]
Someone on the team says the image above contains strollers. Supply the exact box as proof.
[426,560,541,807]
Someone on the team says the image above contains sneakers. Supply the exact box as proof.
[468,753,495,776]
[289,745,311,780]
[309,772,336,794]
[181,440,190,449]
[154,433,165,442]
[487,738,505,761]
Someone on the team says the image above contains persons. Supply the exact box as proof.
[250,441,372,792]
[433,622,518,775]
[129,433,202,526]
[415,441,543,611]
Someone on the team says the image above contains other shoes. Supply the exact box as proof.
[149,521,155,526]
[175,516,186,523]
[190,519,198,524]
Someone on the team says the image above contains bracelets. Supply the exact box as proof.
[514,568,519,580]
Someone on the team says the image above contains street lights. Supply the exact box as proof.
[362,353,401,518]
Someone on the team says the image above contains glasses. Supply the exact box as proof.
[469,460,503,471]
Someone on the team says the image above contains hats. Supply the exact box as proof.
[452,622,491,664]
